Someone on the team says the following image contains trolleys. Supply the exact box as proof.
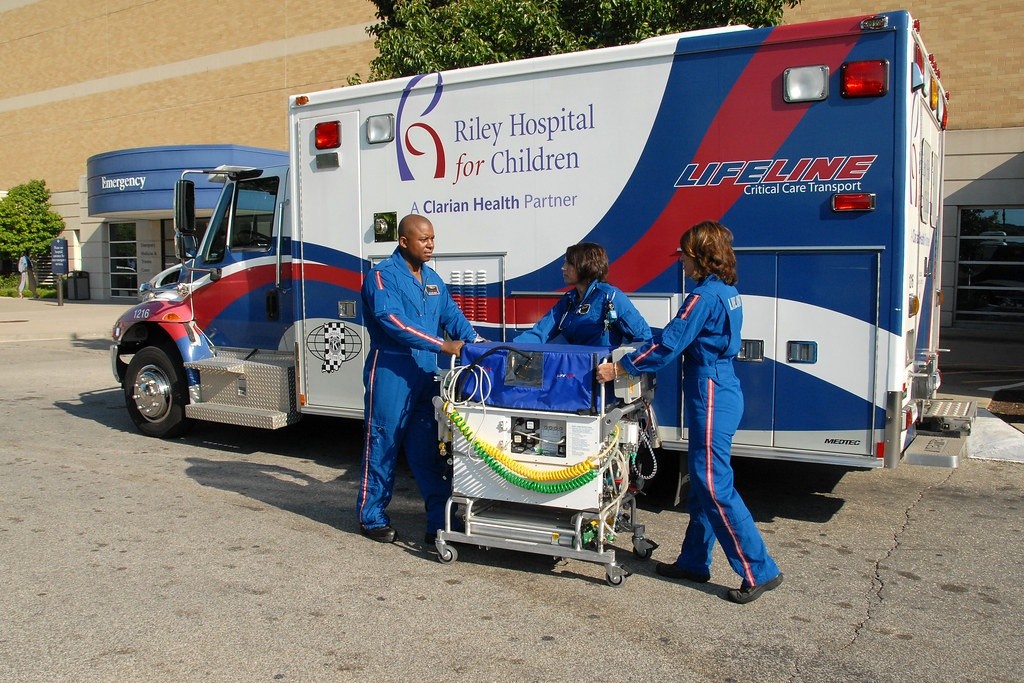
[431,339,659,588]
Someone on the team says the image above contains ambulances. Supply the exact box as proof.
[109,12,976,470]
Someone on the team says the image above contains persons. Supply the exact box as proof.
[596,221,784,604]
[512,243,653,345]
[356,214,493,547]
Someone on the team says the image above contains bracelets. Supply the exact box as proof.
[614,362,620,383]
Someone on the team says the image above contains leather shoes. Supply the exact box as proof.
[425,531,466,546]
[362,523,399,543]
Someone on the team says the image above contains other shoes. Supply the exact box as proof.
[726,573,784,604]
[656,562,710,583]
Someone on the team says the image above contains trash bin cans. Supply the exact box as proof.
[66,270,91,300]
